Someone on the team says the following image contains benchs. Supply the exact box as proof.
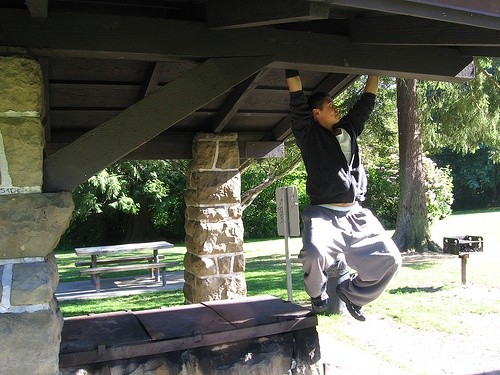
[79,262,175,293]
[75,255,165,283]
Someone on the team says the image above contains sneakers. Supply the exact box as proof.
[335,284,365,322]
[312,271,329,313]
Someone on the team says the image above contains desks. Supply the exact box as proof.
[74,241,174,289]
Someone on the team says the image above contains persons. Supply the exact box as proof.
[285,69,402,321]
